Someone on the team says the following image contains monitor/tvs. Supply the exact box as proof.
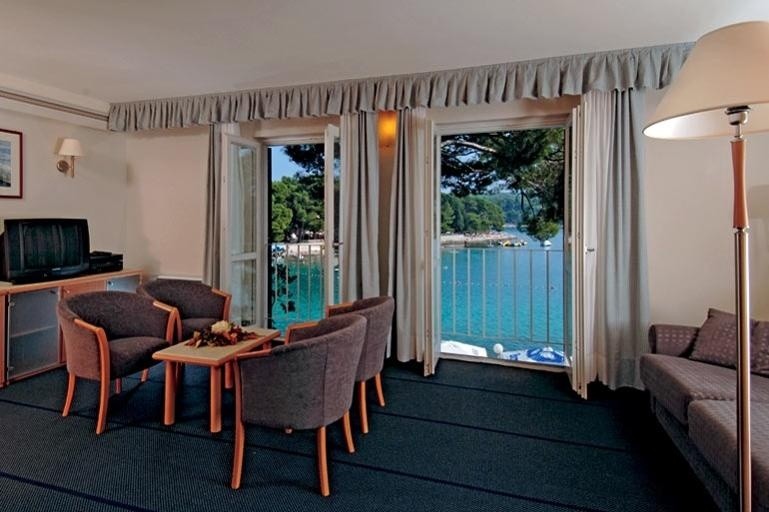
[0,218,89,285]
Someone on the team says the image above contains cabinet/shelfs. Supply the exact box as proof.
[0,269,145,387]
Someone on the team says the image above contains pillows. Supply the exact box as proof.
[743,319,769,374]
[689,308,756,369]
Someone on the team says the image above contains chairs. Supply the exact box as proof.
[58,273,395,498]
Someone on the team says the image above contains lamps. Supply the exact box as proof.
[54,138,84,174]
[643,20,769,512]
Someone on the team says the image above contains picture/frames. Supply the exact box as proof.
[0,128,23,199]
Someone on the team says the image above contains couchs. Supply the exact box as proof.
[642,318,769,512]
